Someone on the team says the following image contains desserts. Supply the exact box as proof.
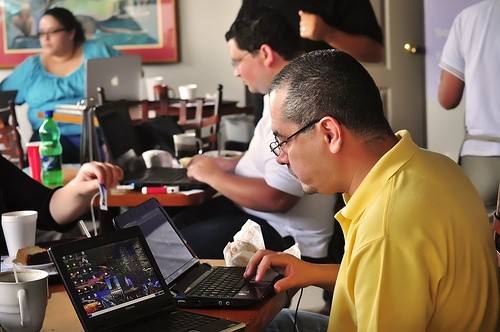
[229,241,258,262]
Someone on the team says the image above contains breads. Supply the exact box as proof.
[15,245,51,265]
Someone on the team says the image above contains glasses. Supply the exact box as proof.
[37,27,67,37]
[269,120,320,156]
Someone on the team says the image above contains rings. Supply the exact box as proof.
[301,26,305,32]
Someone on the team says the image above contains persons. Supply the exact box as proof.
[0,7,138,160]
[434,0,500,214]
[232,0,386,141]
[243,49,499,332]
[187,16,336,261]
[0,147,123,251]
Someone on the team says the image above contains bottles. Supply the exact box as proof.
[39,111,63,189]
[8,98,34,160]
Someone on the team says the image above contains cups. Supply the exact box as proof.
[173,133,202,159]
[179,84,198,101]
[154,84,175,104]
[0,269,49,332]
[25,141,53,183]
[1,210,38,258]
[145,76,163,101]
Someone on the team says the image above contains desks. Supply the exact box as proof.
[94,188,204,207]
[53,102,238,126]
[39,258,300,332]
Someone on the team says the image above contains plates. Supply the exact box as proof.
[3,256,53,267]
[203,151,243,160]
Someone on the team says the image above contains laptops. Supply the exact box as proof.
[56,56,144,111]
[112,197,283,306]
[93,98,207,189]
[47,226,247,332]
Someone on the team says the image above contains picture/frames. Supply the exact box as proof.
[0,0,179,70]
